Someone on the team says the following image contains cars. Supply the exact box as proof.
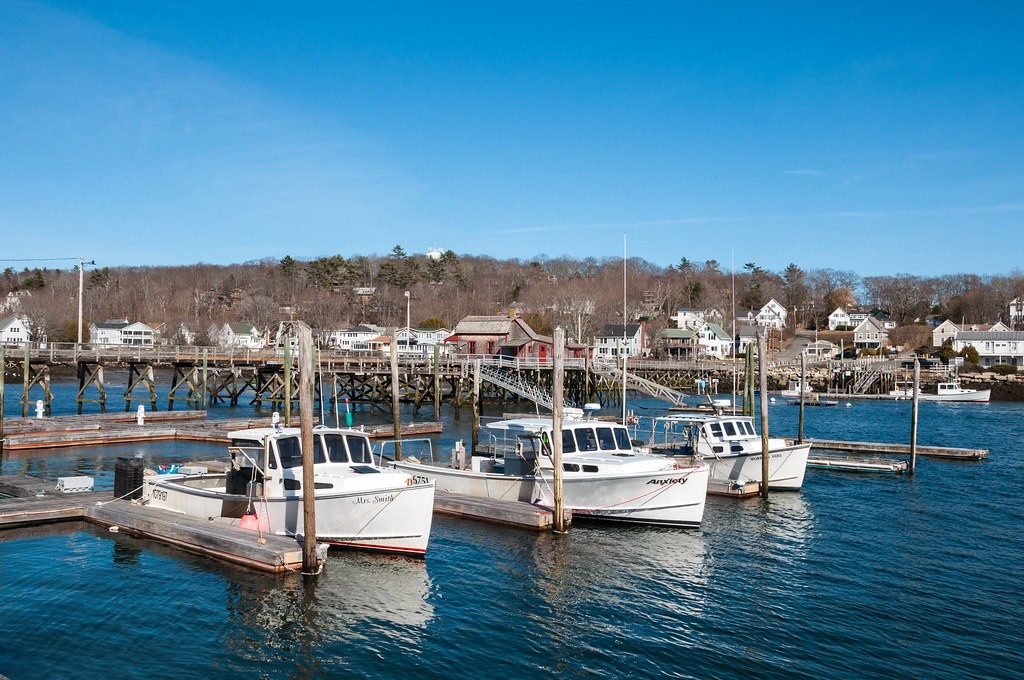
[834,347,860,360]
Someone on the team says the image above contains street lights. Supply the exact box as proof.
[404,290,411,358]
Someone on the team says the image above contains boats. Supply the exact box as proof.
[779,379,813,398]
[142,411,437,560]
[585,244,815,494]
[370,228,714,530]
[917,365,991,403]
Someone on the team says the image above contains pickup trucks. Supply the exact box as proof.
[901,358,933,370]
